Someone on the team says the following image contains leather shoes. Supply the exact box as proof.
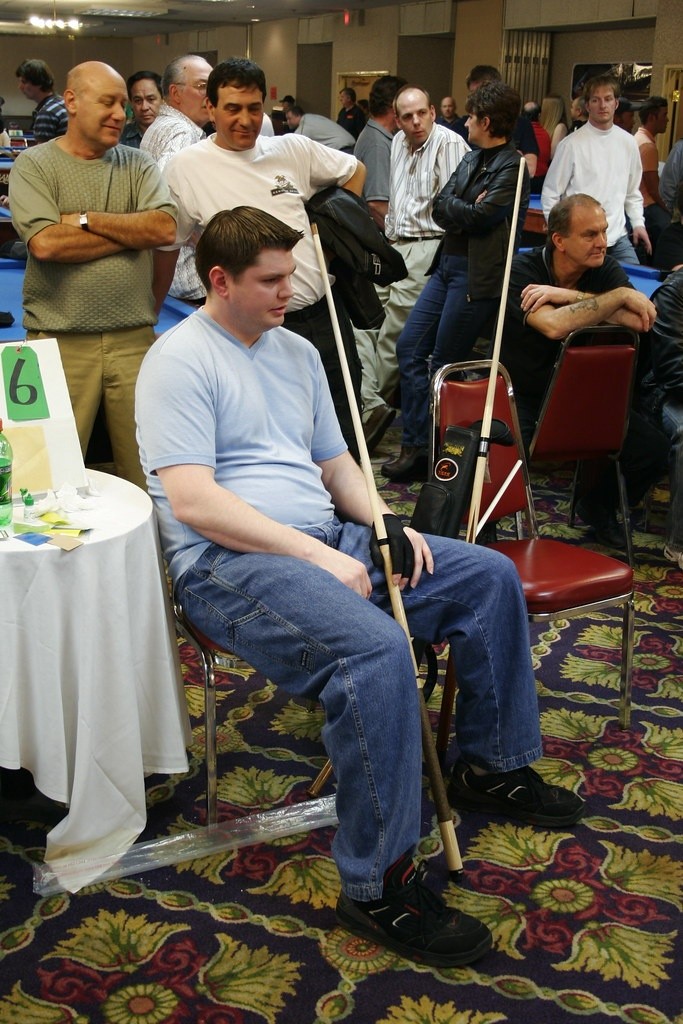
[381,446,429,477]
[362,404,396,450]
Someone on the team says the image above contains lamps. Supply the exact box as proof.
[25,0,83,31]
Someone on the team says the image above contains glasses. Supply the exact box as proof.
[174,82,207,95]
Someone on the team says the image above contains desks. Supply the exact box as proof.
[0,468,193,894]
[10,135,35,147]
[0,259,198,343]
[519,248,662,299]
[523,194,548,235]
[0,205,20,258]
[0,158,14,185]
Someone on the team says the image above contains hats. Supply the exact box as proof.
[279,96,294,103]
[615,98,643,113]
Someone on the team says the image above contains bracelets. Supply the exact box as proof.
[79,211,89,231]
[575,290,585,303]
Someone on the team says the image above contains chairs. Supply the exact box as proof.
[426,359,634,779]
[169,597,243,833]
[529,323,640,572]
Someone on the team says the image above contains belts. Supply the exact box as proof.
[403,236,443,242]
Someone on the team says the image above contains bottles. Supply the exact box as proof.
[0,418,12,528]
[20,487,35,522]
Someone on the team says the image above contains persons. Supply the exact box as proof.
[138,53,216,308]
[10,58,177,494]
[519,92,590,194]
[337,88,368,138]
[0,59,68,207]
[117,69,168,149]
[541,75,655,267]
[135,207,585,964]
[381,79,532,479]
[499,192,683,556]
[437,95,468,143]
[353,75,407,230]
[466,65,540,180]
[350,84,472,458]
[614,95,683,269]
[156,54,368,467]
[277,94,356,154]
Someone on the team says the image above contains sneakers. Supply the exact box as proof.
[445,754,586,828]
[334,852,493,968]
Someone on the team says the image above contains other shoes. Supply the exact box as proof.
[664,546,683,569]
[608,521,627,548]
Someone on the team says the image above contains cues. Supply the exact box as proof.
[428,155,527,792]
[307,221,523,877]
